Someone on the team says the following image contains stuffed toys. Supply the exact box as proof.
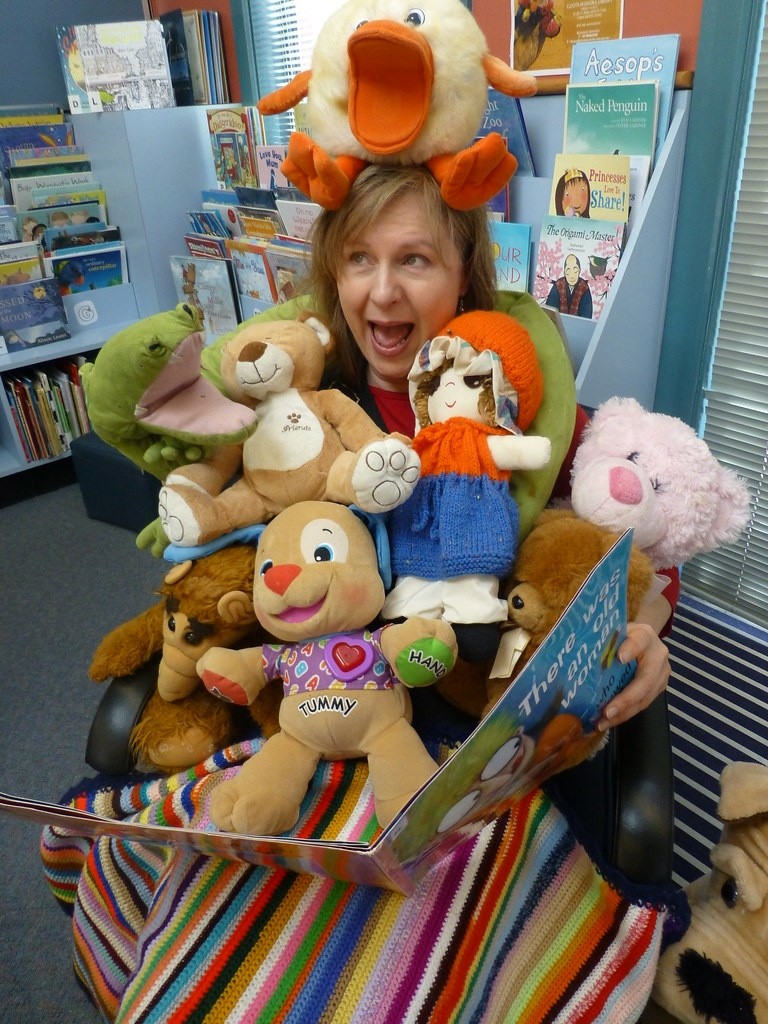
[634,759,768,1024]
[78,294,750,835]
[257,0,536,209]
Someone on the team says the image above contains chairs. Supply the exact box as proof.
[76,628,679,908]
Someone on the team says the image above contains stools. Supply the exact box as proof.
[69,430,161,533]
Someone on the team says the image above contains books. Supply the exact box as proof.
[163,8,681,347]
[1,104,128,460]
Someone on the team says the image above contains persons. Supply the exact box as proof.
[298,166,680,733]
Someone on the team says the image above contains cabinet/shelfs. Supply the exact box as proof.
[507,89,693,412]
[0,318,139,510]
